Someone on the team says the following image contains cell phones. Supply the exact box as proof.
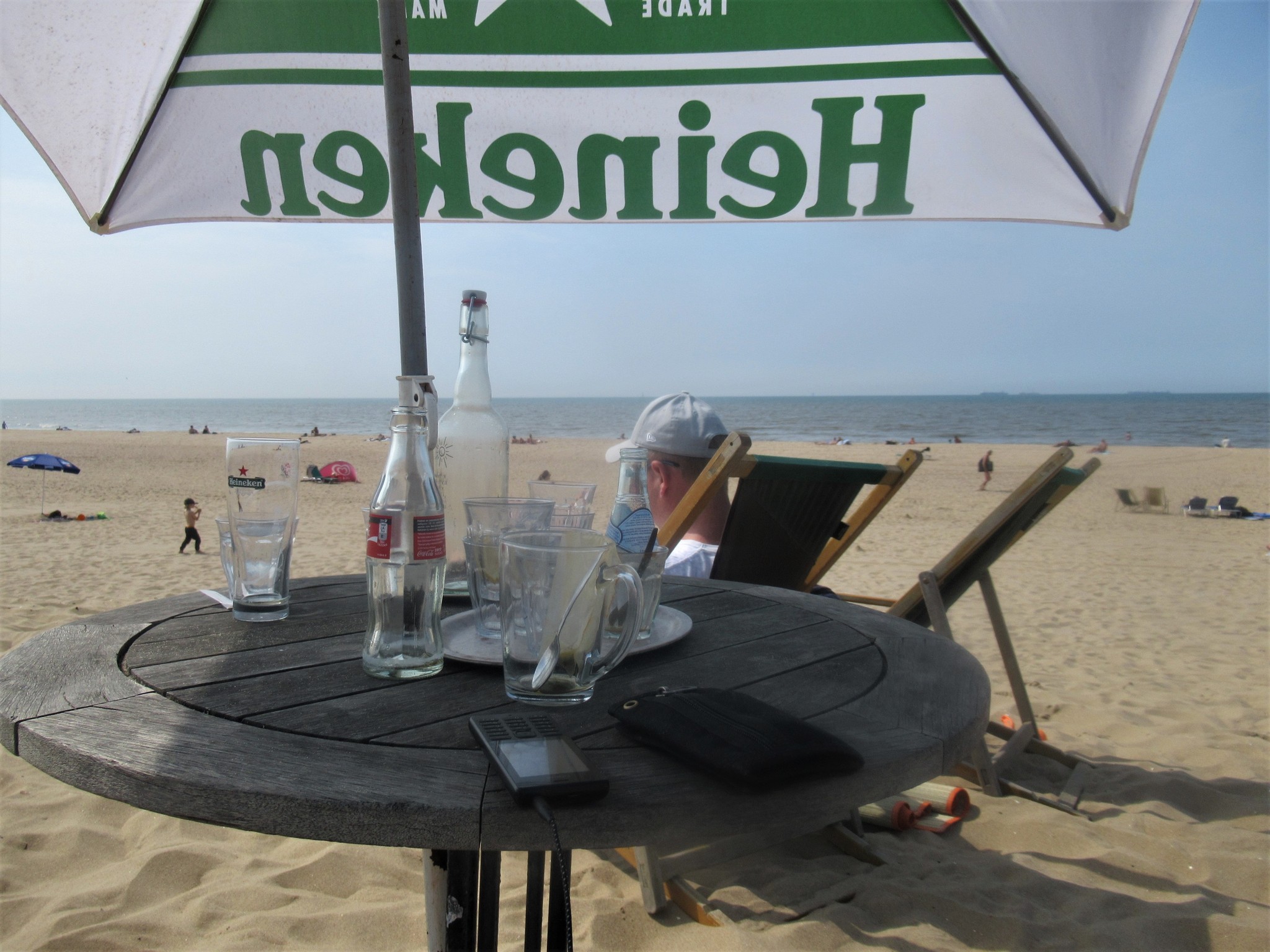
[467,709,608,801]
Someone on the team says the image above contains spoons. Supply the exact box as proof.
[532,542,607,692]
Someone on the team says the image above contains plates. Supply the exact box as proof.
[439,605,693,666]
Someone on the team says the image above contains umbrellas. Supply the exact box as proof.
[6,453,80,514]
[0,0,1205,556]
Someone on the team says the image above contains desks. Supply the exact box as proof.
[0,572,991,952]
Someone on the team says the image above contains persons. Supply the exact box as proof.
[1,420,8,430]
[203,425,211,433]
[512,434,534,444]
[188,425,198,433]
[604,392,733,580]
[311,427,319,435]
[979,450,993,490]
[178,498,203,553]
[1222,437,1234,448]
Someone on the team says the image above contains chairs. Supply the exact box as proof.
[614,430,1098,929]
[1182,497,1242,518]
[1112,486,1169,514]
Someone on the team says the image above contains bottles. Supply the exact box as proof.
[362,405,446,678]
[431,289,510,597]
[605,448,658,552]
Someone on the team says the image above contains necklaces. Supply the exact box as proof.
[1088,438,1108,453]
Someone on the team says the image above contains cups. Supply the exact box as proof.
[363,508,405,595]
[603,545,668,640]
[552,506,594,529]
[464,534,561,640]
[226,437,300,624]
[529,480,597,506]
[215,514,299,601]
[462,498,555,533]
[498,527,643,707]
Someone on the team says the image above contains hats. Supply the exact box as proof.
[604,391,728,464]
[184,498,198,505]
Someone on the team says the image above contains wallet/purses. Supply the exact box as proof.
[609,684,863,797]
